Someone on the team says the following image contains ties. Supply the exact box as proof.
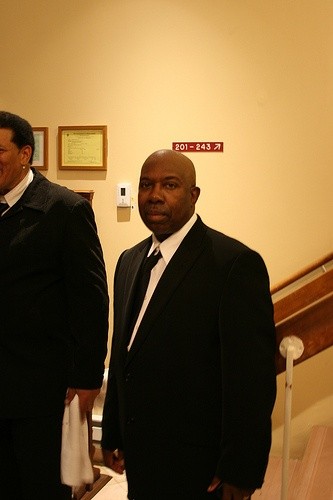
[123,249,161,350]
[0,201,9,217]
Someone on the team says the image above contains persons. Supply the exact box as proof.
[102,150,277,500]
[0,111,109,500]
